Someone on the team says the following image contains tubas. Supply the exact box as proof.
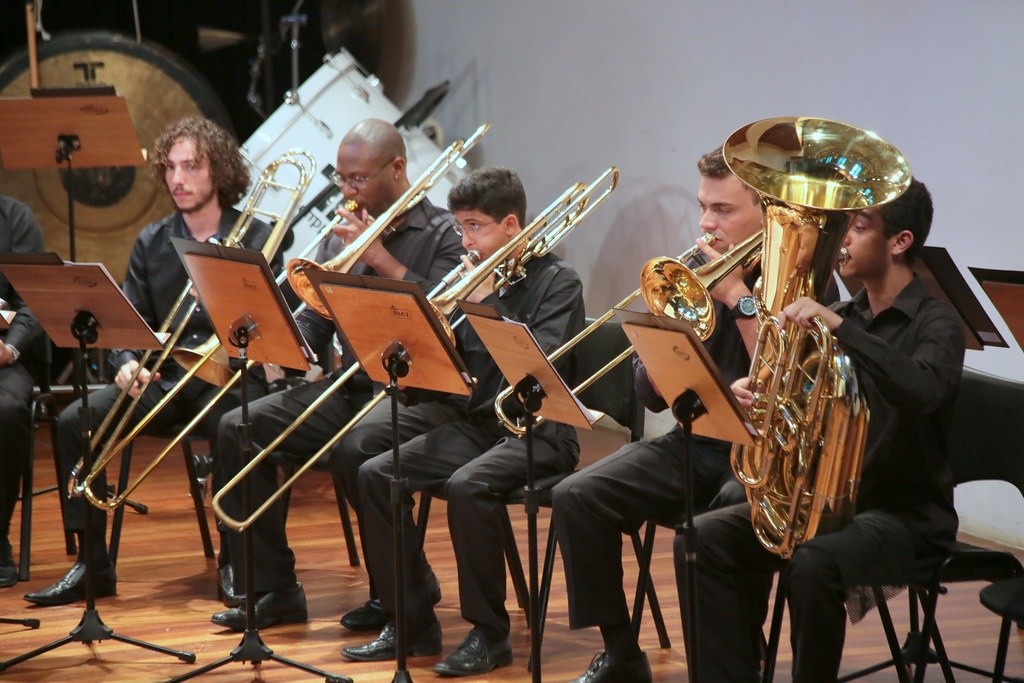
[720,112,915,564]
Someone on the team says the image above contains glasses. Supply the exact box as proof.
[453,220,495,236]
[332,160,393,189]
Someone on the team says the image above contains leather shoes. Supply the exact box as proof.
[0,544,18,588]
[342,621,442,661]
[24,561,116,606]
[217,564,246,607]
[340,599,390,630]
[434,629,513,676]
[574,650,652,683]
[211,582,308,631]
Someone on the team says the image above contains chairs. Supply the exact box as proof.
[20,318,1024,682]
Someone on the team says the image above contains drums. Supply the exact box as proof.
[235,44,471,285]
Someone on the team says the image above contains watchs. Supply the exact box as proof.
[731,296,758,321]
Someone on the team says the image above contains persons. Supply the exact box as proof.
[674,175,970,682]
[211,119,488,633]
[0,194,57,588]
[552,145,840,683]
[23,116,274,606]
[341,164,584,678]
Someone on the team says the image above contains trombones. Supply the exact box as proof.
[210,164,622,535]
[492,226,765,438]
[67,147,319,501]
[82,120,493,514]
